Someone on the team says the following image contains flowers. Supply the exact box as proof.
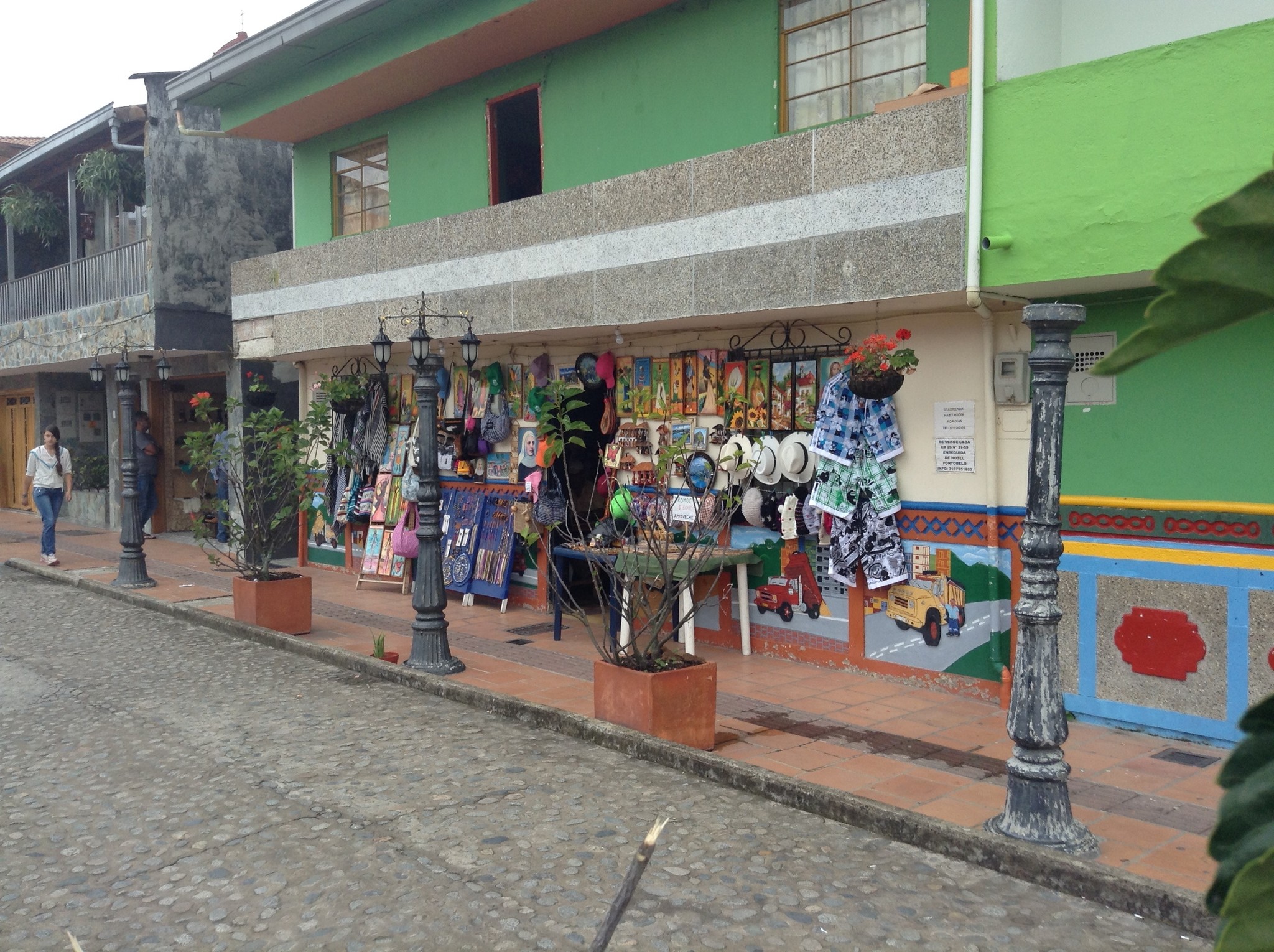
[844,328,916,372]
[184,392,347,569]
[248,372,270,392]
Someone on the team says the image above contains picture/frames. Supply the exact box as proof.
[667,416,697,448]
[603,443,622,469]
[692,428,708,450]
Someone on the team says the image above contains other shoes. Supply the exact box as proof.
[143,534,156,539]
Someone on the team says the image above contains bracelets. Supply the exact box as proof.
[22,494,28,497]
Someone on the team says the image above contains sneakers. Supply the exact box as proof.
[41,553,48,564]
[47,554,59,565]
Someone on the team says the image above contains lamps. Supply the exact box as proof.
[615,328,624,344]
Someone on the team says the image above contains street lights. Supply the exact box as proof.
[87,330,173,588]
[369,288,485,678]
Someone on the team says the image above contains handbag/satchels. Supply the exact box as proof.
[465,403,475,431]
[463,430,481,454]
[404,415,420,468]
[401,464,420,501]
[478,437,491,456]
[481,394,511,444]
[391,502,420,558]
[533,465,567,525]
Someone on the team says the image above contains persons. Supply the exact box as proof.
[21,425,71,566]
[210,430,228,542]
[828,362,840,379]
[134,411,159,539]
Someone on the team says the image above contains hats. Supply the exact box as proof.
[530,353,550,388]
[777,431,816,483]
[485,361,506,395]
[609,487,833,545]
[524,470,542,503]
[597,472,610,502]
[751,435,782,485]
[719,434,751,480]
[535,435,557,468]
[595,351,616,389]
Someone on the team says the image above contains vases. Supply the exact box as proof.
[847,369,906,400]
[247,390,277,406]
[232,571,312,636]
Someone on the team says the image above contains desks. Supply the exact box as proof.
[553,541,756,659]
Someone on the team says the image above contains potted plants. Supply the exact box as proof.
[319,372,368,412]
[514,375,762,751]
[366,631,399,663]
